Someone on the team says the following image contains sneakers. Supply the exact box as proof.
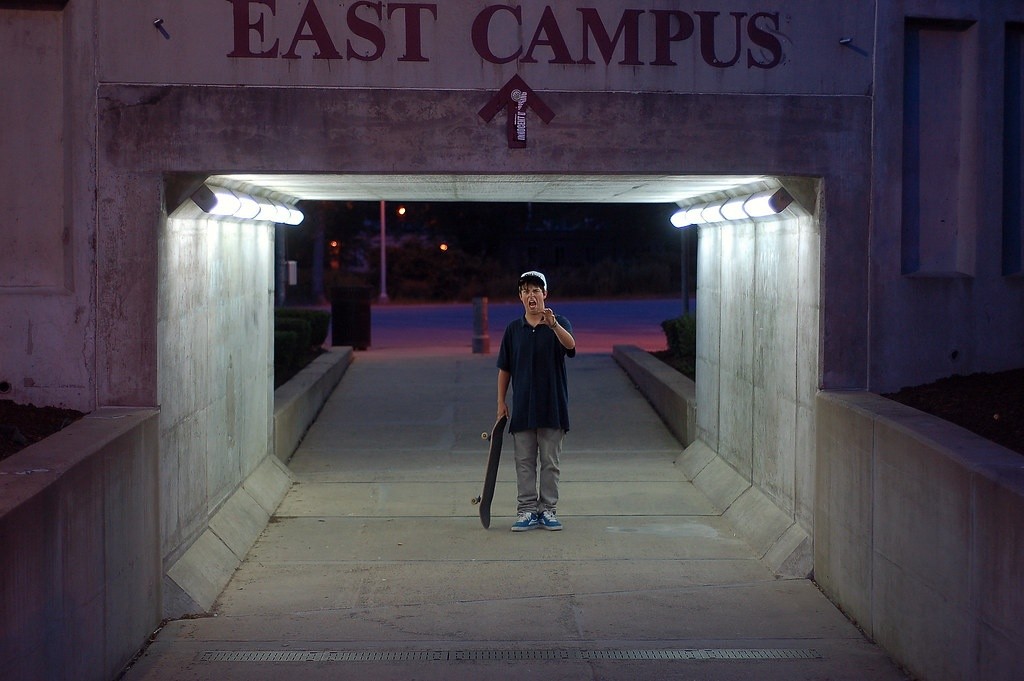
[511,510,562,530]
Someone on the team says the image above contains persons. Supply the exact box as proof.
[496,270,576,533]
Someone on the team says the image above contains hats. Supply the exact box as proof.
[519,270,547,291]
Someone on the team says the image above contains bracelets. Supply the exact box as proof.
[549,321,558,329]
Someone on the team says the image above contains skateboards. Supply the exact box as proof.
[472,414,509,529]
